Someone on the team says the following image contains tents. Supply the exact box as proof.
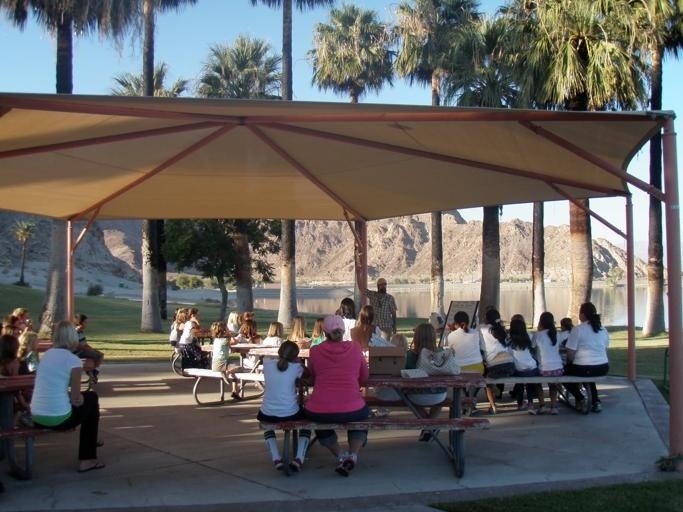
[0,91,683,475]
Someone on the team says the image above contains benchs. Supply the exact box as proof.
[461,376,608,416]
[184,368,265,406]
[275,419,491,479]
[80,359,94,391]
[297,394,477,447]
[0,423,77,479]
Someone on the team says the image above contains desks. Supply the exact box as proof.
[200,344,272,403]
[248,348,309,394]
[0,378,35,467]
[292,373,487,479]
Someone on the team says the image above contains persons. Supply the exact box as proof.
[445,312,484,414]
[238,312,254,327]
[562,303,609,412]
[479,310,516,413]
[351,305,396,357]
[262,322,285,348]
[508,319,538,414]
[357,263,396,343]
[310,318,327,349]
[373,334,409,402]
[337,298,358,342]
[287,316,311,350]
[170,308,207,369]
[73,314,103,387]
[0,335,30,494]
[559,317,573,403]
[236,320,264,370]
[531,311,564,416]
[406,323,448,442]
[256,341,311,473]
[227,312,242,334]
[0,308,32,343]
[18,330,39,375]
[29,320,107,472]
[303,315,370,476]
[210,321,242,401]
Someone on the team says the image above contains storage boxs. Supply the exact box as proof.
[369,347,407,378]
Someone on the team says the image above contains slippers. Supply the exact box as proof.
[77,461,106,472]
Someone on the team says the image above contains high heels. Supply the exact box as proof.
[488,404,496,415]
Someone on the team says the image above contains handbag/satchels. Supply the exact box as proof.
[417,347,462,376]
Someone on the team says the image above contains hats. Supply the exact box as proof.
[376,278,387,285]
[322,315,346,333]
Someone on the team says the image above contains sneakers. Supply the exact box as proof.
[549,408,559,415]
[575,403,582,410]
[431,427,440,442]
[592,401,602,412]
[528,403,537,415]
[221,371,230,385]
[538,405,546,414]
[418,429,431,443]
[335,457,358,478]
[558,394,567,403]
[337,450,358,464]
[580,398,588,413]
[272,456,284,472]
[231,392,243,401]
[518,401,527,411]
[290,457,303,473]
[471,407,478,416]
